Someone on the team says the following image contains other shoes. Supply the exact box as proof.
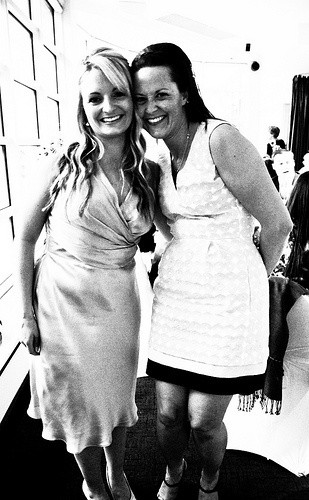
[82,477,110,499]
[154,458,187,499]
[198,476,221,499]
[105,462,136,500]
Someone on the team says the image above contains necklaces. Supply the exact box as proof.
[171,122,193,171]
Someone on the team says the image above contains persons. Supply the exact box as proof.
[131,43,294,500]
[17,47,163,499]
[285,169,309,293]
[267,126,288,160]
[271,149,301,203]
